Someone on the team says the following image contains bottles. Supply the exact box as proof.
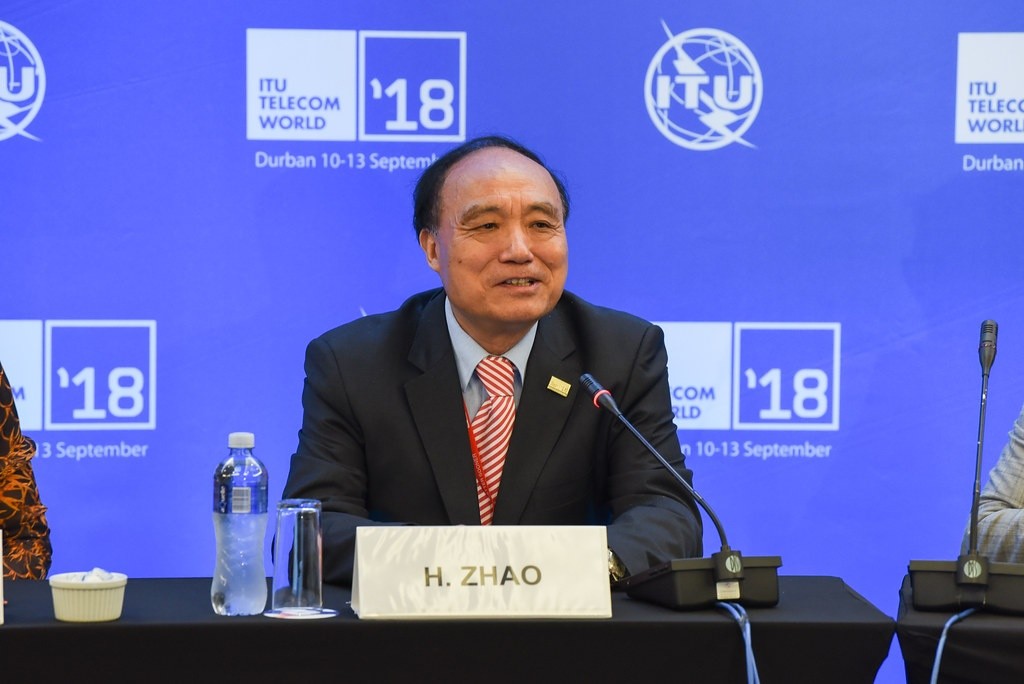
[210,432,268,615]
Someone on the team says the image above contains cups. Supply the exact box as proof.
[272,498,323,615]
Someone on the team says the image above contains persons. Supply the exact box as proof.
[0,361,53,581]
[960,401,1024,564]
[272,133,704,589]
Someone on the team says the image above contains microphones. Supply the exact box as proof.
[908,319,1024,617]
[580,373,783,613]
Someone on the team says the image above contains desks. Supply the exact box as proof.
[0,574,894,684]
[895,572,1024,684]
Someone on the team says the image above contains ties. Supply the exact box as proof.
[468,356,516,526]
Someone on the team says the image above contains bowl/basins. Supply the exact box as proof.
[49,571,128,622]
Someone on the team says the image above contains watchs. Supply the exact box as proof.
[608,548,627,582]
